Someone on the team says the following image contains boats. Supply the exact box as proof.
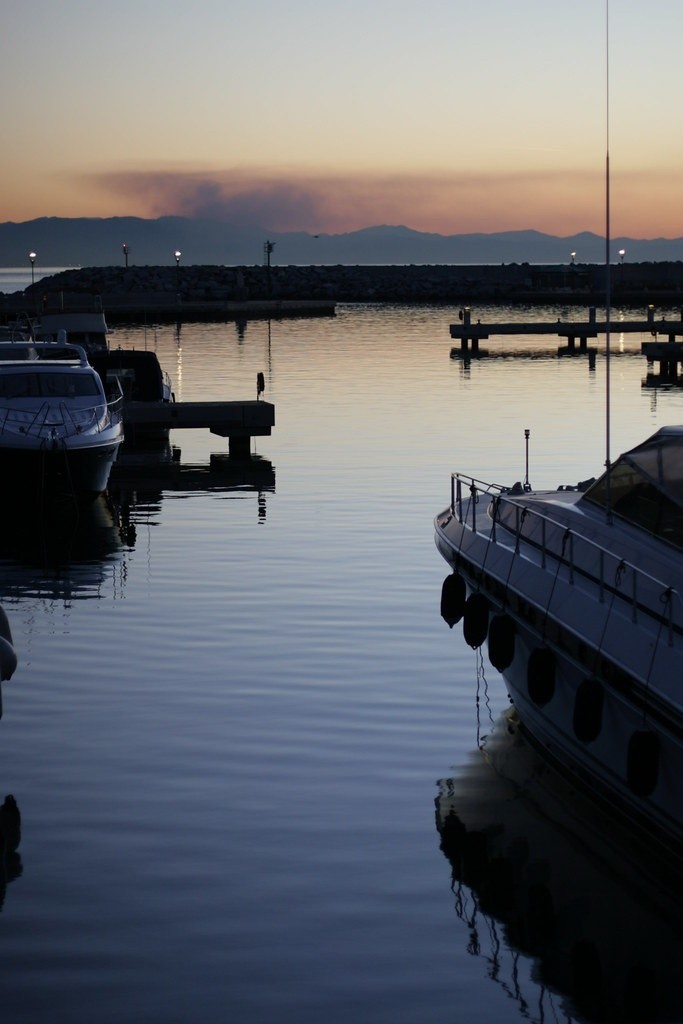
[22,292,108,347]
[1,495,128,568]
[0,329,125,497]
[462,585,683,838]
[431,423,682,723]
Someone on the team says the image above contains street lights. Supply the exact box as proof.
[174,249,182,268]
[571,251,576,263]
[28,252,36,297]
[619,249,625,288]
